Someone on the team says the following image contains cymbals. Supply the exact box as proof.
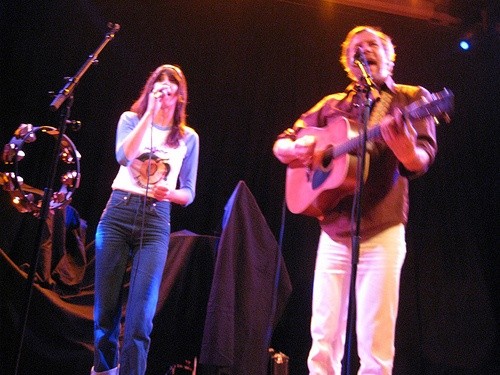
[0,123,82,219]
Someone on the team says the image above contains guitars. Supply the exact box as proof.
[285,87,455,217]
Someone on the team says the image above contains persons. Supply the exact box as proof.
[91,65,200,375]
[272,25,438,375]
[11,197,89,304]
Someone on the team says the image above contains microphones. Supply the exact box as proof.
[355,53,375,87]
[154,92,163,99]
